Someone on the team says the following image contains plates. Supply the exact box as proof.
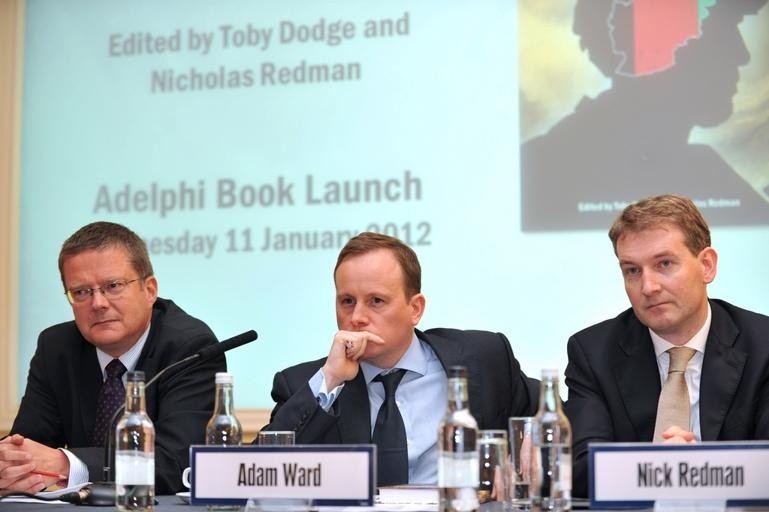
[176,491,192,505]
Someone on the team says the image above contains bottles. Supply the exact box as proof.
[436,366,480,510]
[205,369,243,511]
[114,369,157,510]
[531,369,573,508]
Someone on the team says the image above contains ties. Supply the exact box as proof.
[368,367,408,489]
[94,358,126,449]
[652,345,698,445]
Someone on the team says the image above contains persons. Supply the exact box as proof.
[0,221,226,498]
[521,1,768,228]
[565,195,768,496]
[251,231,531,485]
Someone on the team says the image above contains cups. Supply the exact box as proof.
[476,428,507,504]
[257,429,297,448]
[510,416,542,508]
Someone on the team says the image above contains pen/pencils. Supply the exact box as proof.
[28,469,67,480]
[346,340,351,349]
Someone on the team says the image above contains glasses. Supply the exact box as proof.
[64,274,147,305]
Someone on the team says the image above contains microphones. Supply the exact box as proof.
[79,330,258,507]
